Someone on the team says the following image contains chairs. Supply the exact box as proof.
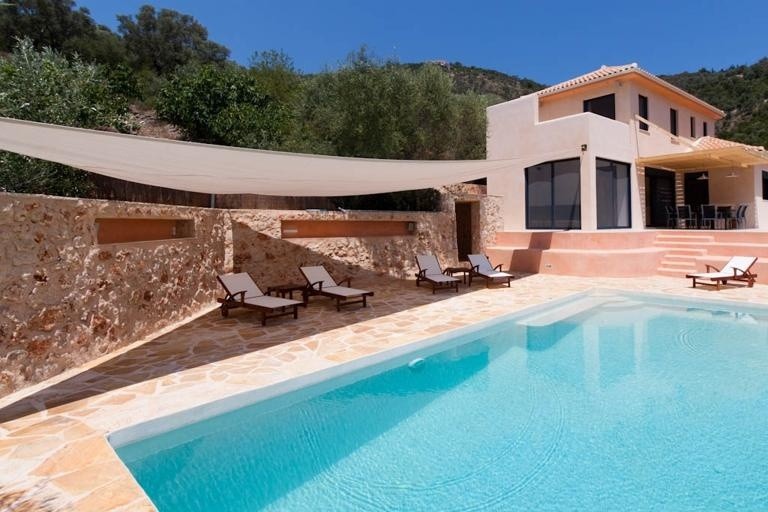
[216,272,304,326]
[686,256,758,291]
[415,254,461,294]
[299,265,373,312]
[463,253,514,288]
[664,204,749,229]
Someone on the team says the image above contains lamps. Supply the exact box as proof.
[724,162,739,178]
[696,164,712,180]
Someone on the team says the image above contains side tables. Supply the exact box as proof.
[447,267,472,287]
[267,283,307,313]
